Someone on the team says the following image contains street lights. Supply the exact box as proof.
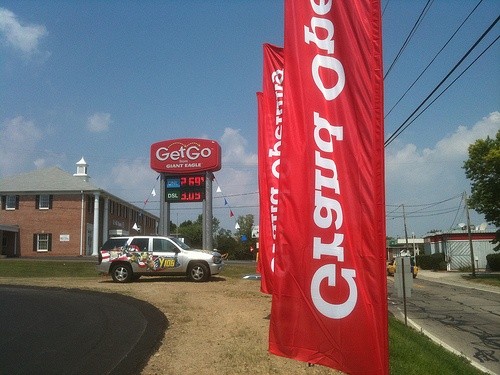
[411,232,416,266]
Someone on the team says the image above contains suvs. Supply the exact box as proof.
[99,235,222,283]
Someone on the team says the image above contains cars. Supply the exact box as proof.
[386,257,415,278]
